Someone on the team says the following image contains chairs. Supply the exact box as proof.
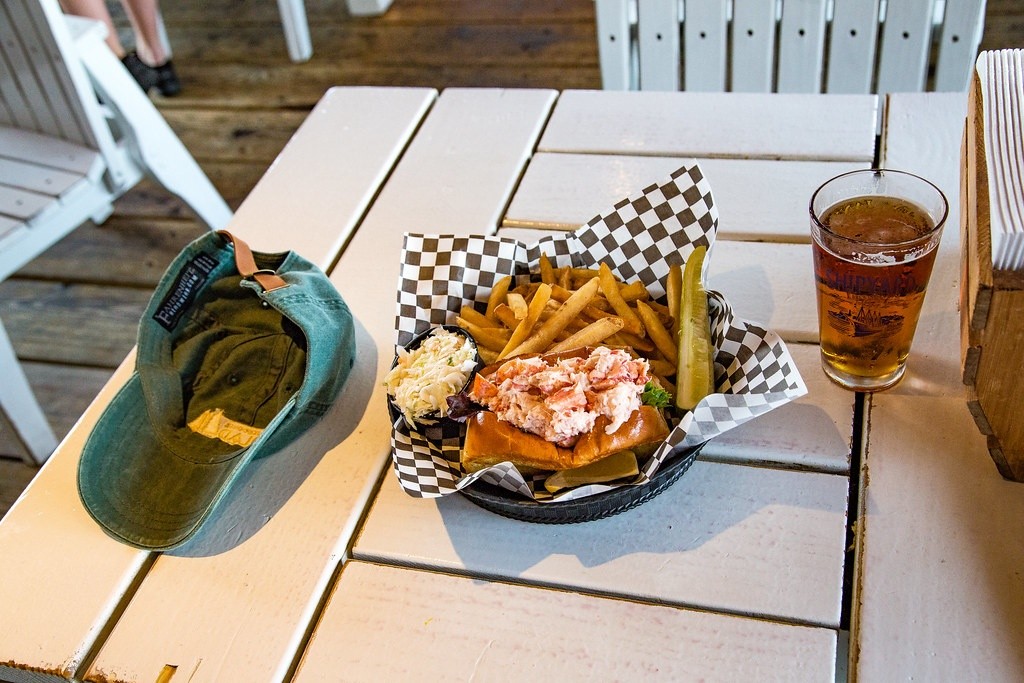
[595,0,987,136]
[0,0,235,464]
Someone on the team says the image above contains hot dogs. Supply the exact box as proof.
[445,344,671,478]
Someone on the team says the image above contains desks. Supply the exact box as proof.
[0,85,1023,682]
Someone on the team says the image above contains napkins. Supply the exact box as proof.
[976,48,1024,272]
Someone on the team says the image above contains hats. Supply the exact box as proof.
[76,228,357,553]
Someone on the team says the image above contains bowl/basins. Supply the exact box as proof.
[390,325,484,431]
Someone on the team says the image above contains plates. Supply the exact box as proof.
[387,356,710,526]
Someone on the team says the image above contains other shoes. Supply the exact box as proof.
[123,50,158,93]
[156,61,182,96]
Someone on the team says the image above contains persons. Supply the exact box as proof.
[58,0,179,104]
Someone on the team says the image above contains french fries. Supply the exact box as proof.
[452,254,676,397]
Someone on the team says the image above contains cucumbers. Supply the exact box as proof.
[676,245,716,409]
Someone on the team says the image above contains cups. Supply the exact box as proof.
[809,169,948,393]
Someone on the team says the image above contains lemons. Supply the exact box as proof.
[544,450,639,493]
